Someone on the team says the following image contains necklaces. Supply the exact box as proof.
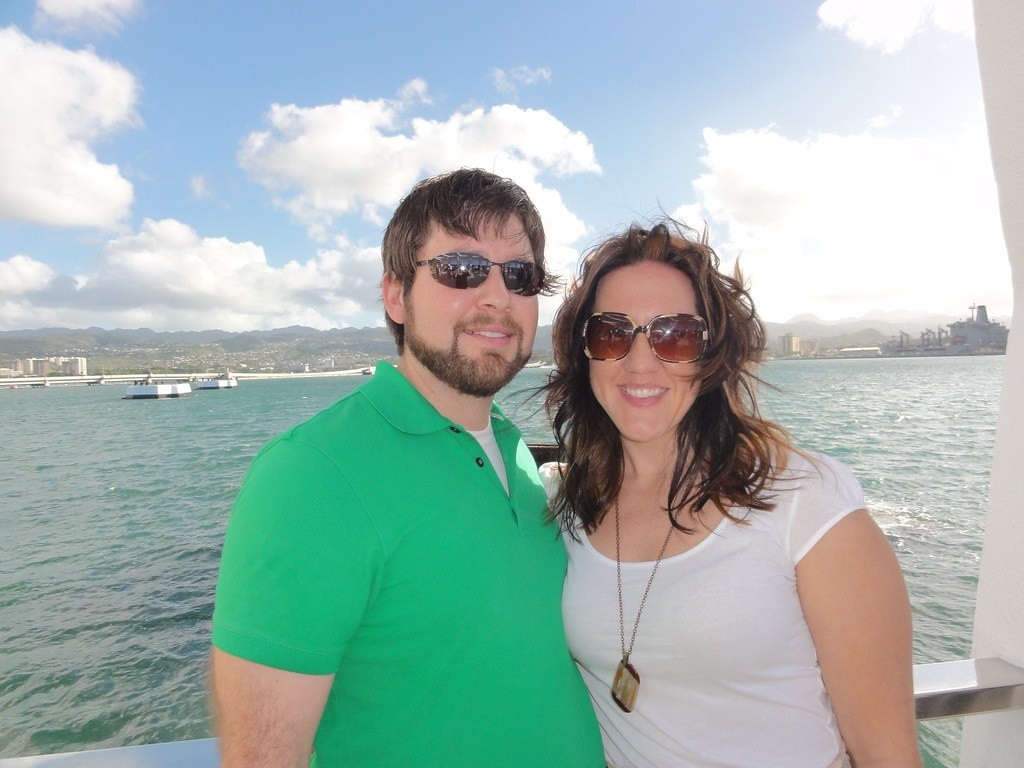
[609,460,697,714]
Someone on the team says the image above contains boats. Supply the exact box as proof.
[523,361,546,368]
[197,372,238,389]
[125,381,193,399]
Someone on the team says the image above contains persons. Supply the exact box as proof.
[209,165,609,768]
[536,223,920,767]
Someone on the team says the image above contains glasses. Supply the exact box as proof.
[583,312,707,363]
[415,250,546,298]
